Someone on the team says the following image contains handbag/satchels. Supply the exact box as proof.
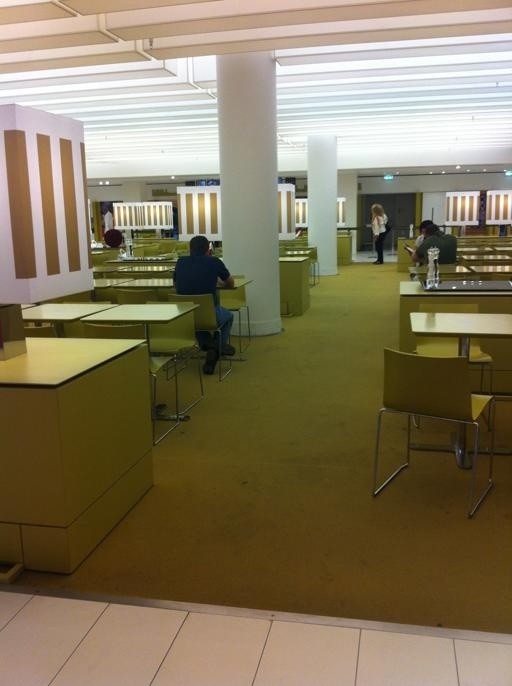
[386,221,393,234]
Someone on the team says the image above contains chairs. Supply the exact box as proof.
[372,348,495,518]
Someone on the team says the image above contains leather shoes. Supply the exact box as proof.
[203,346,218,374]
[221,343,236,355]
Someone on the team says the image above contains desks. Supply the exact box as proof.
[398,236,511,469]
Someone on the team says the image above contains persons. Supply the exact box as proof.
[173,235,235,376]
[412,219,434,266]
[410,223,458,267]
[370,203,389,265]
[102,207,113,236]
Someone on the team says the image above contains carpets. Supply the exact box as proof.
[2,261,512,636]
